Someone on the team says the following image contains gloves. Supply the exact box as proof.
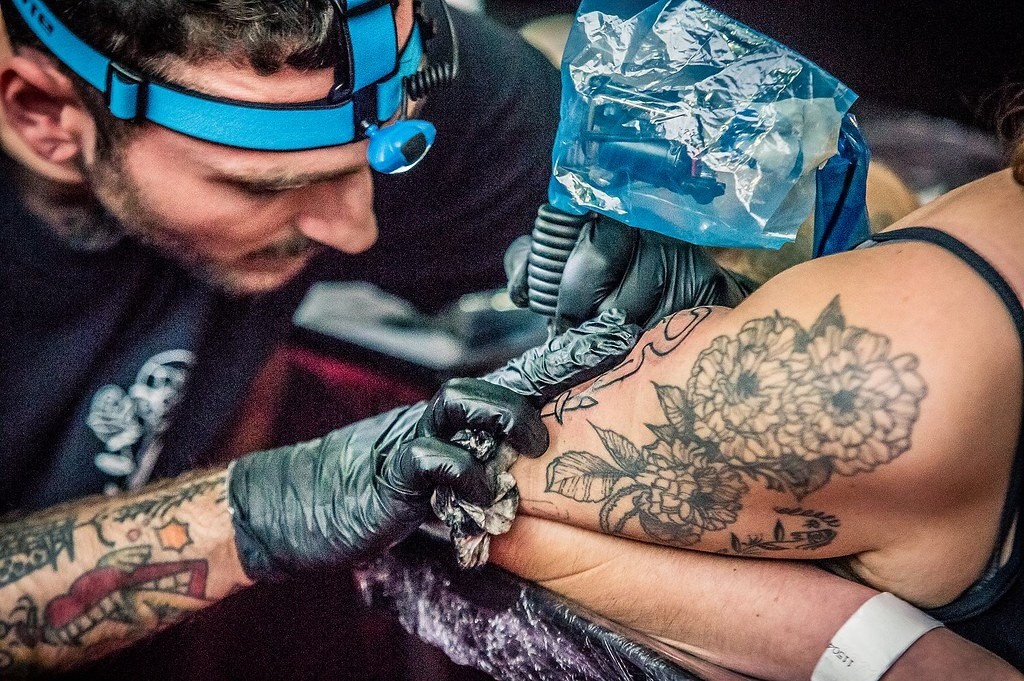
[503,212,760,339]
[225,307,645,584]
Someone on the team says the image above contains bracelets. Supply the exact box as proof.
[807,591,945,681]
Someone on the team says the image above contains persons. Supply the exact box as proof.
[0,0,766,680]
[490,79,1023,681]
